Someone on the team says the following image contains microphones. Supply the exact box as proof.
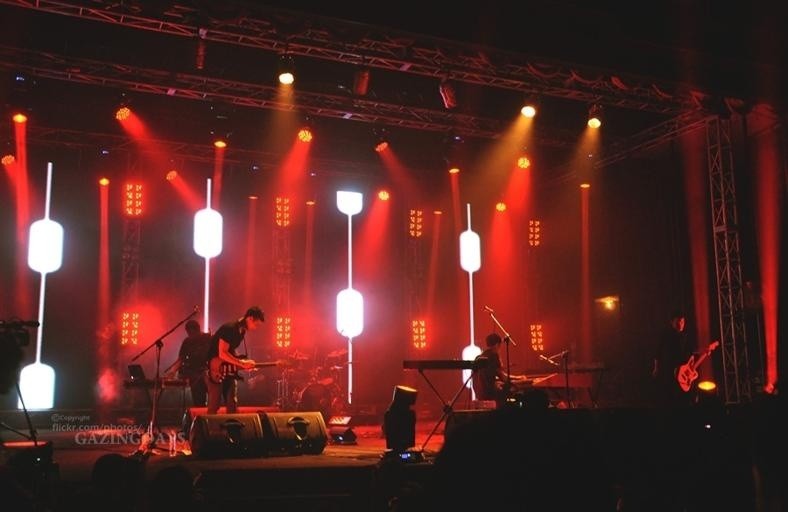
[538,354,555,365]
[483,304,494,313]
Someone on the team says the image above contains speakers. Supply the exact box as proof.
[262,411,328,455]
[188,413,264,458]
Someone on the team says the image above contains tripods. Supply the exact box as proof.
[129,310,196,459]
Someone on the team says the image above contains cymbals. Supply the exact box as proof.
[328,349,347,357]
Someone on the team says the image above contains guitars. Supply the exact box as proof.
[677,341,719,392]
[209,354,288,384]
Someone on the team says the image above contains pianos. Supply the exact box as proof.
[403,360,498,368]
[495,373,595,407]
[124,379,190,387]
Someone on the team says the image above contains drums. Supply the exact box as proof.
[291,382,331,420]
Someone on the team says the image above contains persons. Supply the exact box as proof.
[650,309,712,404]
[203,306,266,415]
[470,332,528,409]
[0,449,201,509]
[162,320,213,407]
